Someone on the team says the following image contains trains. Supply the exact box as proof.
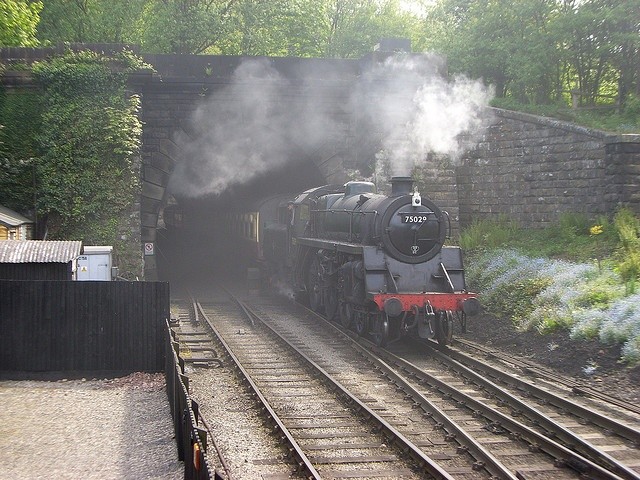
[163,177,475,347]
